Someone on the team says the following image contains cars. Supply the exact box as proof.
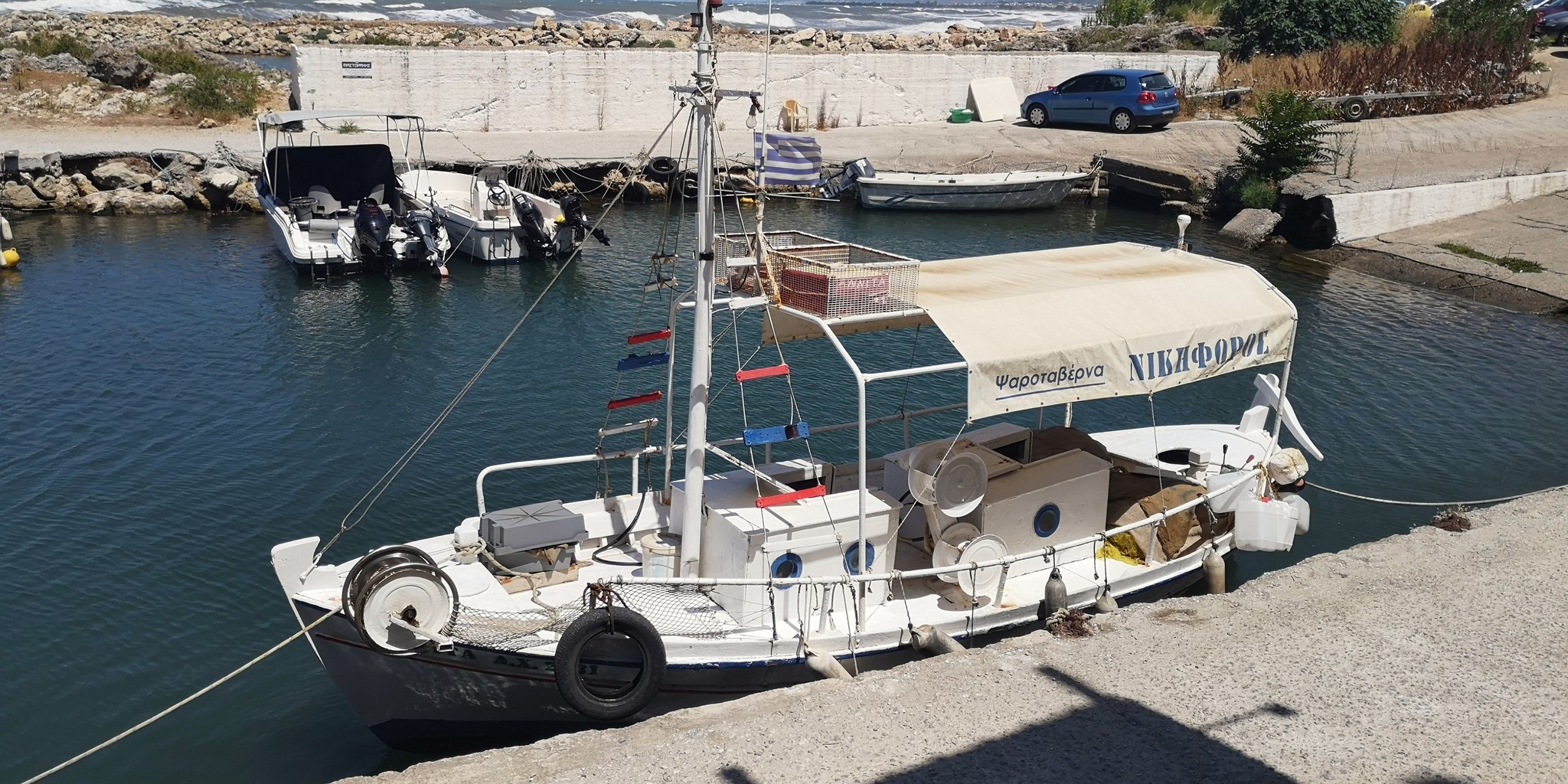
[1506,0,1568,31]
[1535,11,1568,47]
[1423,0,1445,10]
[1020,69,1181,134]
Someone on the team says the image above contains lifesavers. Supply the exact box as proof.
[600,158,699,203]
[554,606,667,722]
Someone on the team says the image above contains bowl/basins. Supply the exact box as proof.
[950,109,974,123]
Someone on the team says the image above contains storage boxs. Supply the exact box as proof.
[478,499,590,576]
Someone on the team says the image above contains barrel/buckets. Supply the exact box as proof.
[640,533,682,578]
[1206,470,1310,552]
[289,197,319,220]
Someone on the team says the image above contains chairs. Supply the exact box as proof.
[306,185,342,219]
[348,183,385,218]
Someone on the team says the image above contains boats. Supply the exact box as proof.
[811,157,1088,211]
[269,0,1323,763]
[395,163,611,265]
[254,109,451,279]
